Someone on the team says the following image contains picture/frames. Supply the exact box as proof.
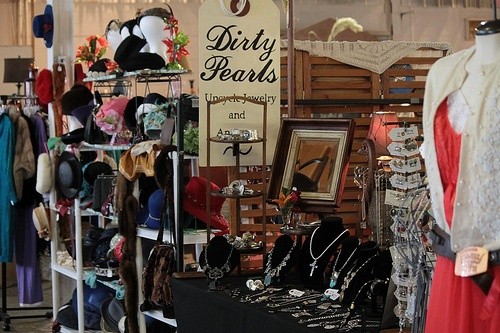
[264,117,355,207]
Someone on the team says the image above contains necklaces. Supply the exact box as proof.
[204,227,389,333]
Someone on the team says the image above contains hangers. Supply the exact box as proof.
[0,94,43,120]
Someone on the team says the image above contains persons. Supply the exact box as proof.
[198,236,239,284]
[107,8,180,66]
[422,19,500,333]
[263,217,393,311]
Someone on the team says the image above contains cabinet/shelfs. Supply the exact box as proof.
[205,96,267,274]
[46,67,229,332]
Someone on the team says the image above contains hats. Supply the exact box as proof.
[35,68,52,104]
[34,153,54,196]
[32,4,53,48]
[56,83,228,333]
[31,203,50,242]
